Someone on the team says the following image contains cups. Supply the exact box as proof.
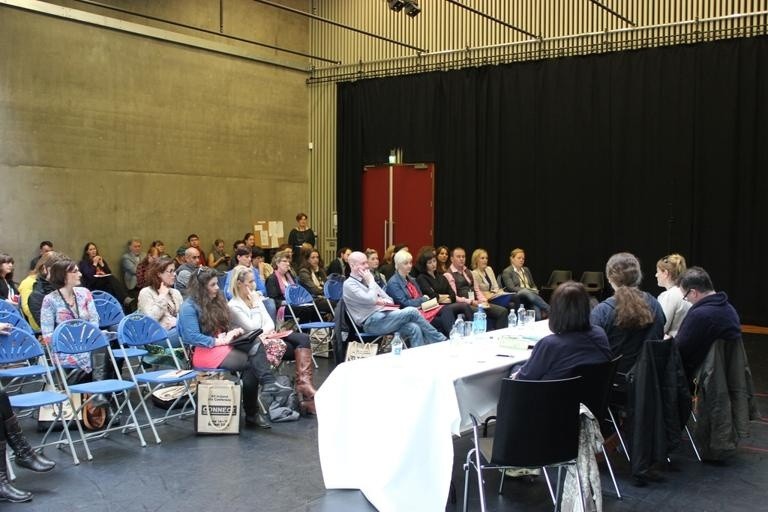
[527,310,535,323]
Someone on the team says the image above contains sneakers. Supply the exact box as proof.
[499,468,540,477]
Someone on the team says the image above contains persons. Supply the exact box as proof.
[0,254,17,300]
[138,258,318,429]
[119,233,351,329]
[342,246,551,347]
[656,254,693,340]
[18,238,125,424]
[496,280,612,484]
[288,213,315,248]
[589,252,666,373]
[0,323,56,503]
[674,266,742,344]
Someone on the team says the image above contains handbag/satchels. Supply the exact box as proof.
[310,327,329,357]
[152,380,241,435]
[38,383,83,432]
[229,329,262,345]
[345,341,378,361]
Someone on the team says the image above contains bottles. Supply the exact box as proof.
[508,309,517,328]
[449,304,487,342]
[391,332,402,357]
[517,304,526,325]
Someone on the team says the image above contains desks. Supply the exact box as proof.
[314,320,555,511]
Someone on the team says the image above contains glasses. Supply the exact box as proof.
[197,266,207,276]
[683,290,690,301]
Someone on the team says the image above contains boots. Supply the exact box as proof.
[91,353,120,425]
[0,411,56,502]
[294,348,316,414]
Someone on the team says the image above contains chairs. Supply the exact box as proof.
[581,352,631,502]
[461,376,593,512]
[580,271,605,302]
[612,338,703,480]
[684,337,747,465]
[541,270,572,303]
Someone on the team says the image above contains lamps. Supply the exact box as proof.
[386,0,421,19]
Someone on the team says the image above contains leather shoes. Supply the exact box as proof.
[261,381,294,396]
[245,413,271,429]
